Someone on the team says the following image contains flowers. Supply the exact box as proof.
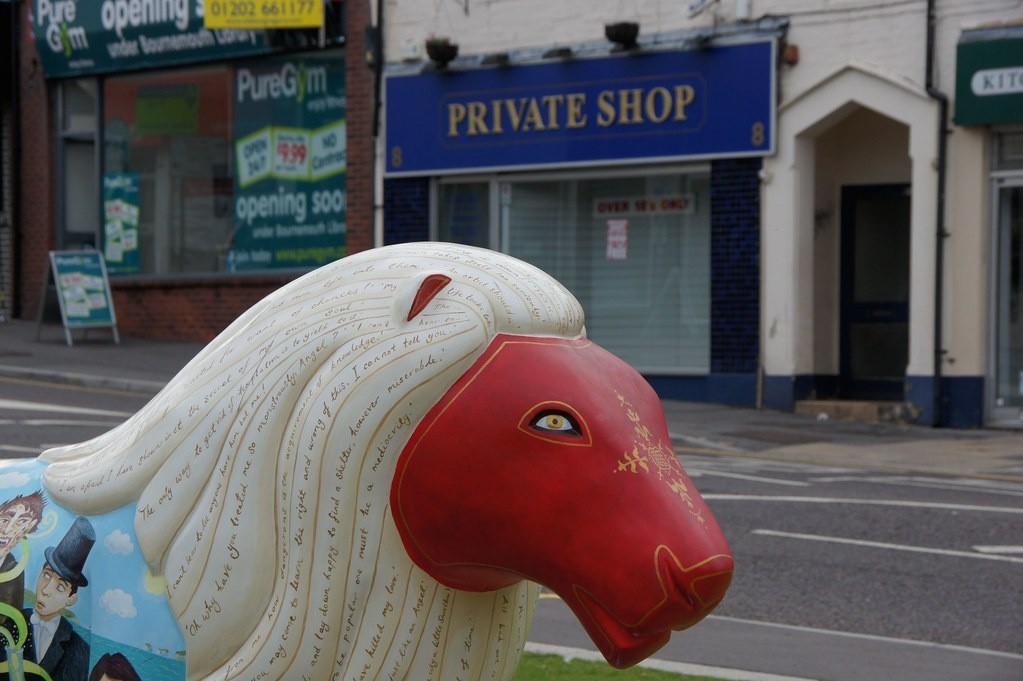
[428,32,450,46]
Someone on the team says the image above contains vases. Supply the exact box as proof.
[426,41,458,61]
[604,23,639,42]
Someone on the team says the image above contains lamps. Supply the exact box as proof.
[543,49,568,58]
[481,55,506,64]
